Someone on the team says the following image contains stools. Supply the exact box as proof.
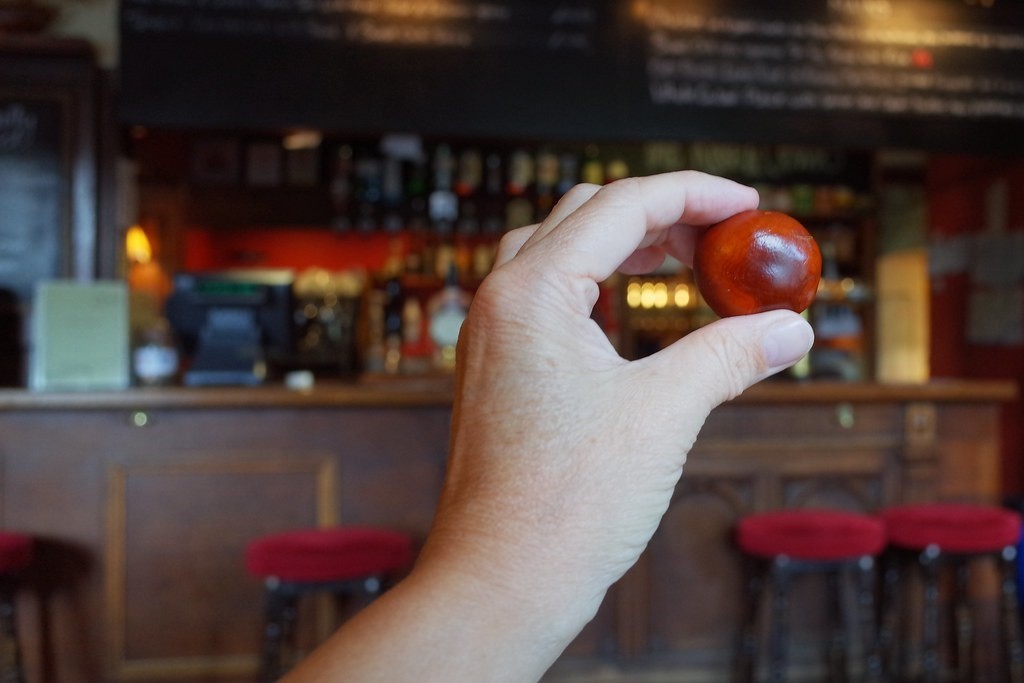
[733,499,1024,683]
[247,524,422,683]
[0,530,40,682]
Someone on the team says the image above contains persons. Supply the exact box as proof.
[271,170,815,683]
